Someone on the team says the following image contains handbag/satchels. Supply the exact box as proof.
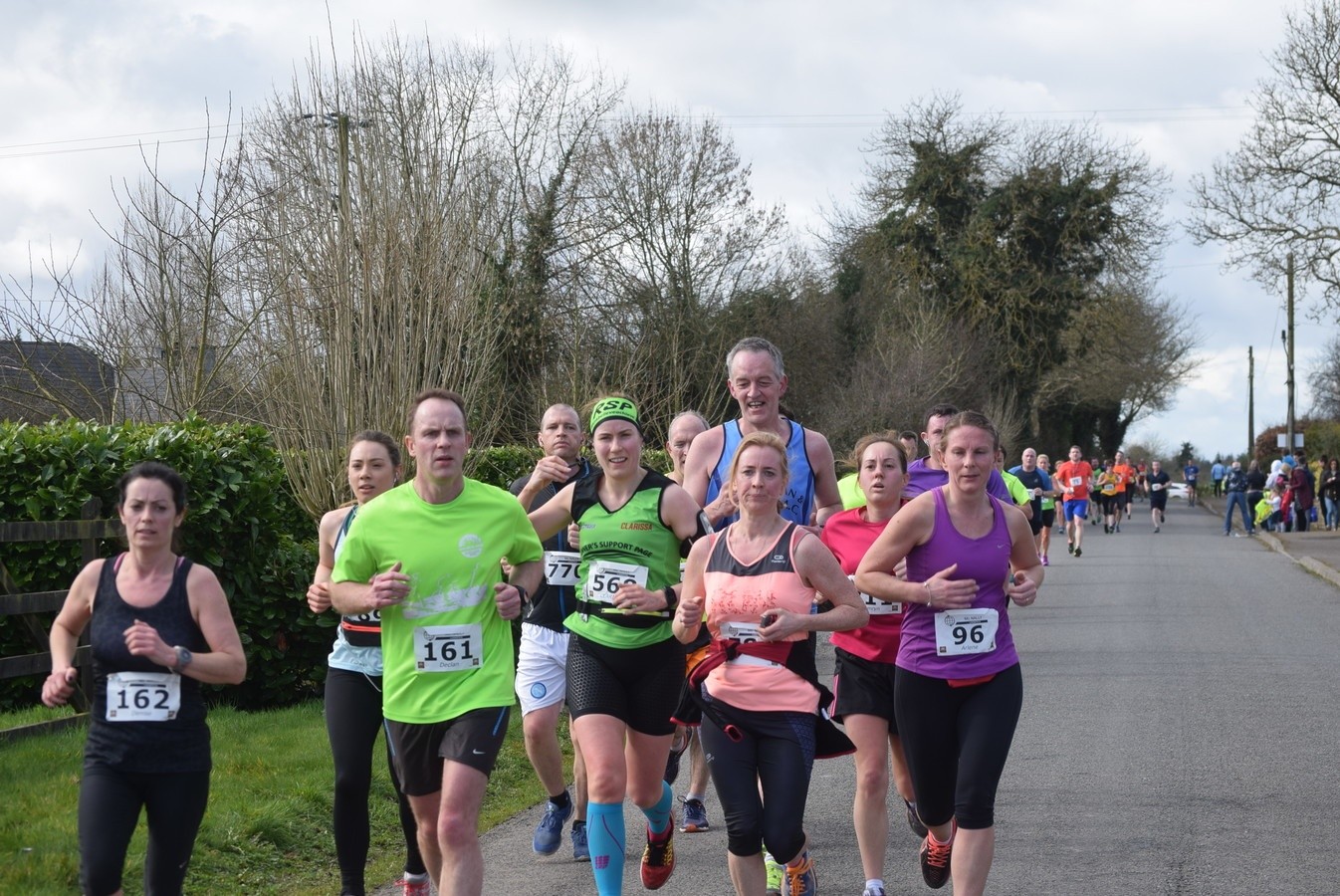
[1309,506,1318,523]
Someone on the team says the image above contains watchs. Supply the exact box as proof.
[513,585,530,612]
[657,585,677,613]
[168,644,192,673]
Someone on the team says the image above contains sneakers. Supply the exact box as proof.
[862,887,885,896]
[570,822,591,861]
[762,843,784,896]
[532,791,574,856]
[397,877,431,896]
[677,795,709,833]
[639,809,676,891]
[782,850,818,896]
[904,799,929,838]
[919,815,958,889]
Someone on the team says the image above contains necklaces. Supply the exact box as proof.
[731,517,777,563]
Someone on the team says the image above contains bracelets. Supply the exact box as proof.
[1042,491,1045,496]
[922,581,933,607]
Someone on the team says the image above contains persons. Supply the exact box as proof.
[306,335,1340,896]
[41,462,247,896]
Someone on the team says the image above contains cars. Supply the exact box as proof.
[1166,483,1196,500]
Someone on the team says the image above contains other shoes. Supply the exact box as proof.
[1154,527,1160,533]
[1041,556,1049,566]
[1038,553,1040,558]
[1068,537,1075,553]
[1188,501,1194,506]
[1160,514,1164,523]
[1223,530,1229,536]
[1074,546,1082,557]
[1059,507,1132,534]
